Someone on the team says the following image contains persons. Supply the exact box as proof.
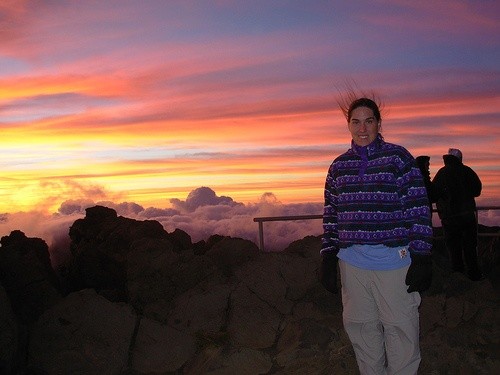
[320,92,434,374]
[434,149,481,299]
[405,155,430,284]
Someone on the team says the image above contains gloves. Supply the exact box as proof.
[320,252,342,296]
[404,250,431,292]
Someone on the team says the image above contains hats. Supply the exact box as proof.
[448,147,462,161]
